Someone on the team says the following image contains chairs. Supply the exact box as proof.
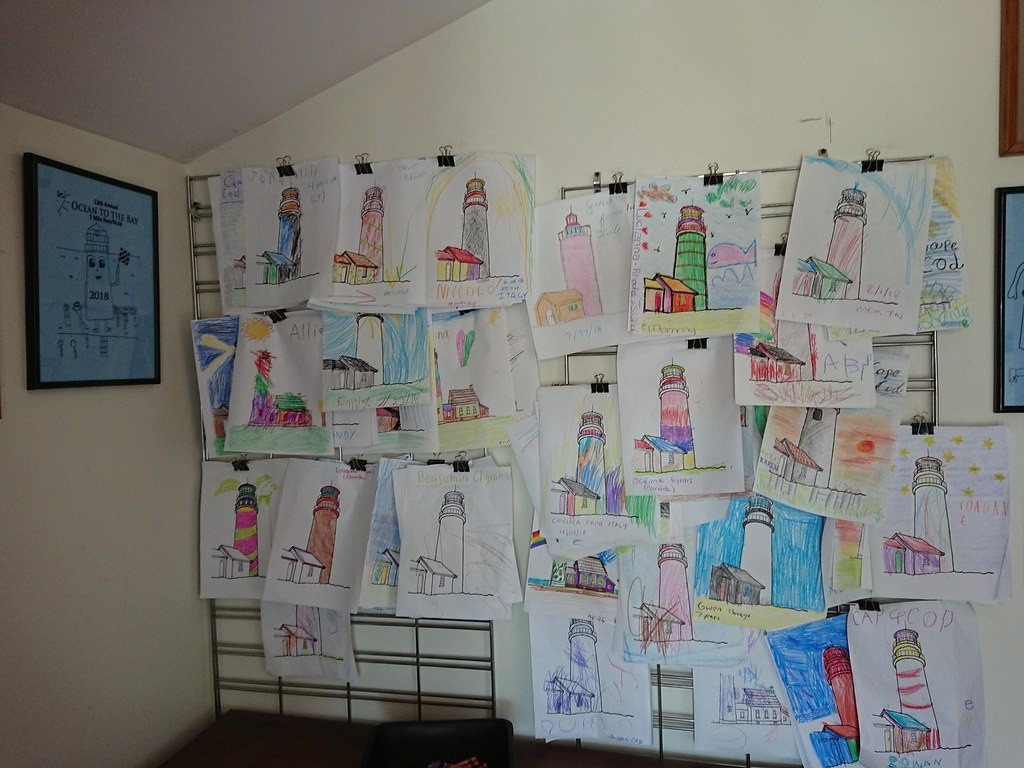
[359,718,513,768]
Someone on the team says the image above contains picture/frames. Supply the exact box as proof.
[22,153,160,391]
[999,1,1023,157]
[992,186,1024,414]
